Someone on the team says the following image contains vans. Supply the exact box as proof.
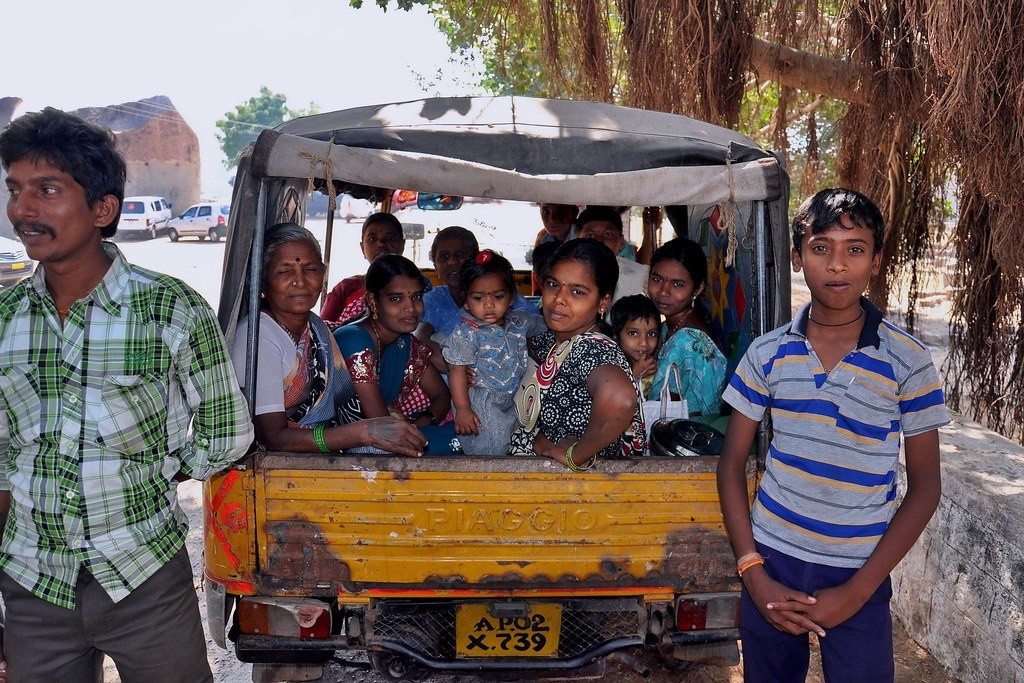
[115,194,173,239]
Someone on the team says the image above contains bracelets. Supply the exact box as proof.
[313,423,331,454]
[565,441,597,472]
[736,552,765,577]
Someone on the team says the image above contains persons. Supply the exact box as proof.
[717,188,953,683]
[0,106,255,683]
[233,203,726,472]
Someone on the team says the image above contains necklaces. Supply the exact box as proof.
[823,367,831,372]
[586,323,596,332]
[808,306,864,328]
[57,309,68,314]
[670,309,692,340]
[370,318,382,357]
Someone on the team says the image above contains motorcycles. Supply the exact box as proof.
[197,93,792,682]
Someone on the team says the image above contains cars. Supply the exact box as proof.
[0,234,33,286]
[165,201,232,242]
[308,190,381,222]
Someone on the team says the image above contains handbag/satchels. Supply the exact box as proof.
[642,363,688,457]
